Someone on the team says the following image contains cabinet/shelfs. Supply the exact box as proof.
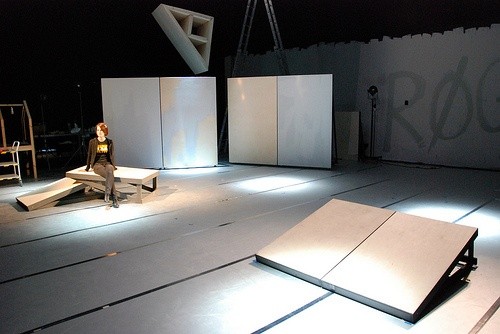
[0,141,23,188]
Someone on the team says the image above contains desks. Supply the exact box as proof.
[65,163,160,205]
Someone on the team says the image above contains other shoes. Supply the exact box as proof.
[113,199,119,208]
[105,194,110,203]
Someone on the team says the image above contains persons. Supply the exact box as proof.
[85,123,119,208]
